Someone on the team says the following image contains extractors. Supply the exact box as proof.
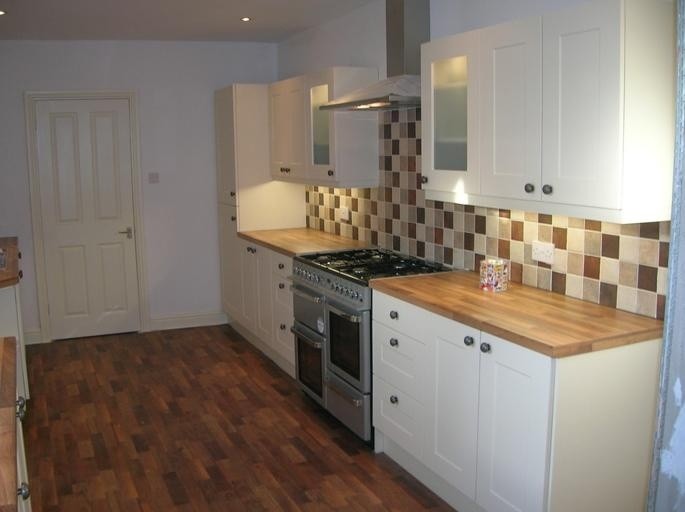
[317,3,431,113]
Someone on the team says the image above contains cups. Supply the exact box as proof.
[481,259,509,293]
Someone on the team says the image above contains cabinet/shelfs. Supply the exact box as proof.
[271,249,295,380]
[481,0,678,225]
[266,74,303,184]
[214,84,307,206]
[425,316,664,512]
[302,67,380,189]
[218,204,270,359]
[373,289,424,483]
[420,28,481,206]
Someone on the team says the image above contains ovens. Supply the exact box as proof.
[293,277,378,442]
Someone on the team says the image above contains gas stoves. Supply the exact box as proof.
[294,247,453,300]
